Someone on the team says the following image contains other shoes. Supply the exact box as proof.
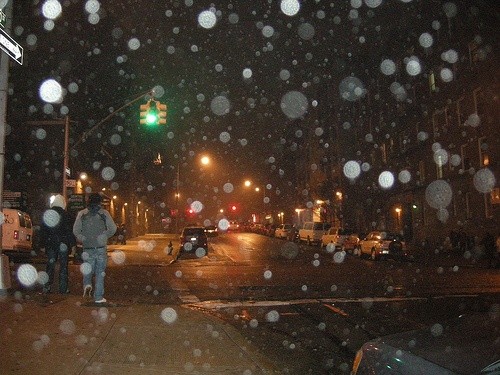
[95,297,107,304]
[82,284,91,299]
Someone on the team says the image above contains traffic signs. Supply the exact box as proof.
[0,28,24,66]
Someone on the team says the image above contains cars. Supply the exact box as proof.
[349,319,500,375]
[226,220,293,240]
[204,224,218,237]
[321,226,356,252]
[340,232,368,257]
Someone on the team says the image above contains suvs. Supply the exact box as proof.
[356,229,410,264]
[178,225,209,255]
[298,221,332,247]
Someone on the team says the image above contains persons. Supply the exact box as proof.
[120,223,127,246]
[73,191,117,303]
[484,232,500,269]
[451,226,466,251]
[37,194,76,294]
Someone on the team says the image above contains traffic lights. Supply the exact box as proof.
[155,101,168,126]
[138,103,150,126]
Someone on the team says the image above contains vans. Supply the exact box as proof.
[2,206,34,253]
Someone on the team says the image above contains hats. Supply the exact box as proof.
[87,193,101,206]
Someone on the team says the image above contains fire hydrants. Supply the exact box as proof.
[167,240,173,255]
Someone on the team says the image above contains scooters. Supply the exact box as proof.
[286,230,301,243]
